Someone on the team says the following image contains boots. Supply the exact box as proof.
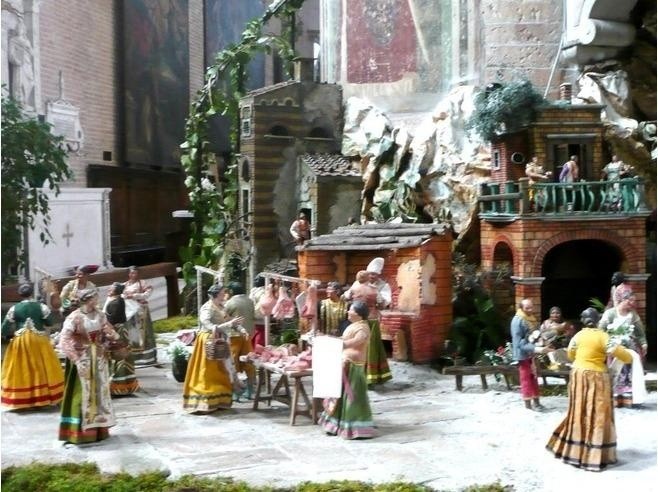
[525,399,532,410]
[533,398,543,409]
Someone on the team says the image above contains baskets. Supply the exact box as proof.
[108,339,129,360]
[205,326,230,359]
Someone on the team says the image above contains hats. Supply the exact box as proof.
[366,257,384,274]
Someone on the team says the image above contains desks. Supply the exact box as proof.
[238,354,314,426]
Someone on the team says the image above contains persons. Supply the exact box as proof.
[524,155,553,212]
[58,287,121,446]
[546,307,633,471]
[181,211,394,440]
[605,271,634,312]
[102,282,141,397]
[59,263,102,323]
[541,306,577,348]
[509,298,558,413]
[2,283,65,411]
[37,275,66,333]
[599,153,635,211]
[597,285,649,408]
[120,266,157,366]
[559,154,588,211]
